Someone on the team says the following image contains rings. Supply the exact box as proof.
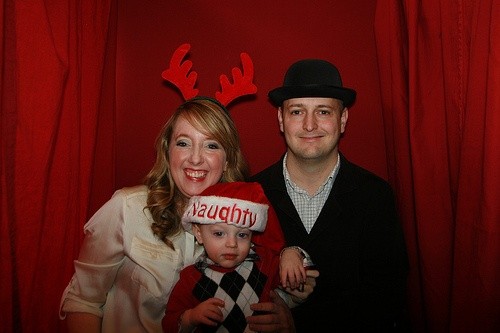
[298,282,304,292]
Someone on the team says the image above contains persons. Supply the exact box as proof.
[57,94,321,333]
[244,58,410,333]
[160,180,315,333]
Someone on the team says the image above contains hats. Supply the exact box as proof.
[268,58,356,106]
[181,182,286,289]
